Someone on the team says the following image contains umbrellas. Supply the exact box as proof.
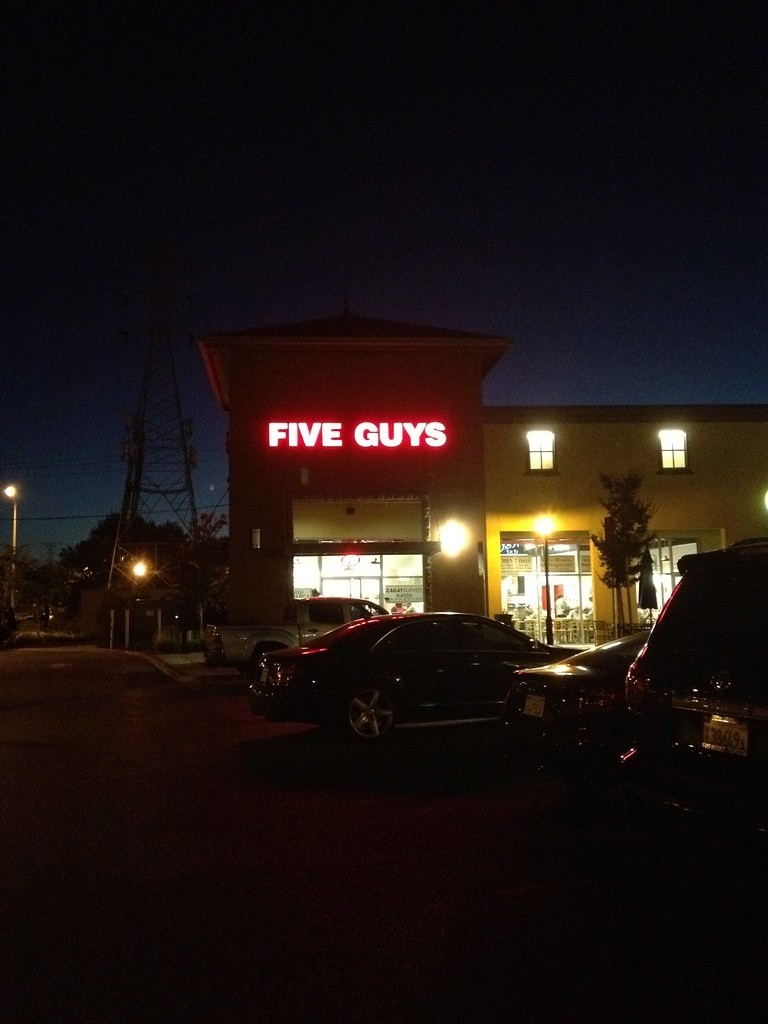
[638,547,658,629]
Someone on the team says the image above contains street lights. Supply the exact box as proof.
[530,512,557,648]
[0,481,21,616]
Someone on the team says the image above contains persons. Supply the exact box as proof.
[6,607,18,647]
[567,605,586,618]
[312,589,322,597]
[526,605,537,619]
[585,595,593,617]
[391,602,415,614]
[556,595,570,617]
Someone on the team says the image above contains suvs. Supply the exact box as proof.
[610,537,767,850]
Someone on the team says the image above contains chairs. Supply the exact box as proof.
[514,618,594,645]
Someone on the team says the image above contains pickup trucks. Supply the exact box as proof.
[204,597,390,683]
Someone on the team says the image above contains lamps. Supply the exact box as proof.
[252,528,261,549]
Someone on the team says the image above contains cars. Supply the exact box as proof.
[496,630,653,756]
[246,610,585,743]
